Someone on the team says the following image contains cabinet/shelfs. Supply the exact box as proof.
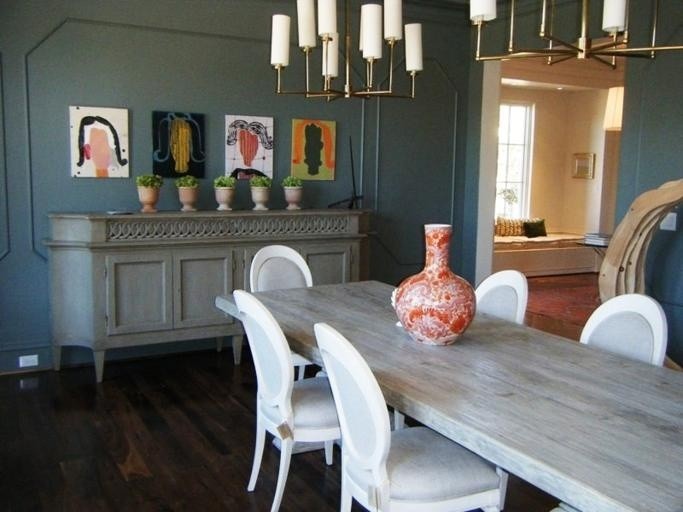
[42,211,368,382]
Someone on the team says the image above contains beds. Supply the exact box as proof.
[492,232,596,278]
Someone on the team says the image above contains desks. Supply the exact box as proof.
[215,279,683,512]
[575,239,608,260]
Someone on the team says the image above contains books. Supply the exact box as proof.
[584,232,609,246]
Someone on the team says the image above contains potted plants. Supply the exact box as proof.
[175,175,201,212]
[214,176,237,211]
[281,175,303,211]
[136,174,163,214]
[248,174,271,211]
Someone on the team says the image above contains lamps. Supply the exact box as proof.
[269,0,424,100]
[469,0,683,70]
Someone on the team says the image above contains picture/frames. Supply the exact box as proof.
[69,106,130,179]
[570,151,596,180]
[292,118,336,182]
[224,114,274,180]
[152,110,205,179]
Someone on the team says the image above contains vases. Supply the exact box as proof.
[389,224,477,347]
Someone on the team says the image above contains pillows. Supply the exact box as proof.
[525,219,547,238]
[496,217,525,236]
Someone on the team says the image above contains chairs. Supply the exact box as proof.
[250,244,314,380]
[580,293,669,370]
[311,321,509,512]
[232,290,342,512]
[473,268,528,323]
